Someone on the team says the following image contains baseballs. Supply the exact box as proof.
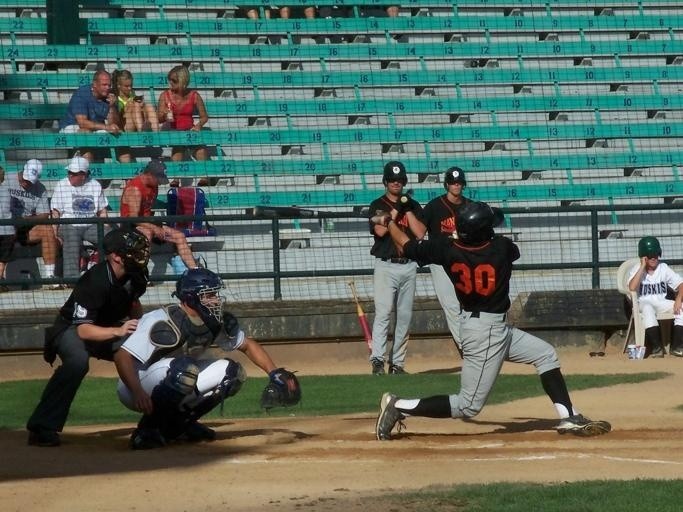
[402,197,408,203]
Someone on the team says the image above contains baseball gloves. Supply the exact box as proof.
[261,368,300,407]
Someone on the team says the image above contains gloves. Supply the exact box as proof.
[271,368,300,405]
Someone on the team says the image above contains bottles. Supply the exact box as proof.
[164,100,174,123]
[324,218,335,233]
[627,344,636,360]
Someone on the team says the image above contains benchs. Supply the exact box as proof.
[0,0,683,305]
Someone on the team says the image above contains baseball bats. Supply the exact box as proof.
[253,206,376,217]
[348,282,371,353]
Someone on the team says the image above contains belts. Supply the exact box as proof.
[471,312,480,317]
[381,258,408,264]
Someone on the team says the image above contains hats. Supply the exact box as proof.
[66,157,89,173]
[447,167,464,184]
[23,158,42,184]
[149,161,169,184]
[385,161,406,182]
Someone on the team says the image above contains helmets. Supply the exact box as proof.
[176,268,226,332]
[457,203,493,244]
[104,229,151,266]
[639,237,661,257]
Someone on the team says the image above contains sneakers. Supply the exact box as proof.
[178,421,215,442]
[558,414,611,434]
[131,431,162,449]
[376,393,405,439]
[29,431,60,447]
[653,347,683,357]
[373,360,401,375]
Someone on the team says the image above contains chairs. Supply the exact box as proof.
[615,257,677,359]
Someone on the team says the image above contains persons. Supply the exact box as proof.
[114,267,301,449]
[369,162,423,375]
[121,159,197,287]
[629,236,683,357]
[59,64,209,187]
[0,147,5,182]
[241,7,402,43]
[0,159,70,291]
[26,226,152,446]
[50,156,114,289]
[370,202,611,441]
[423,166,476,357]
